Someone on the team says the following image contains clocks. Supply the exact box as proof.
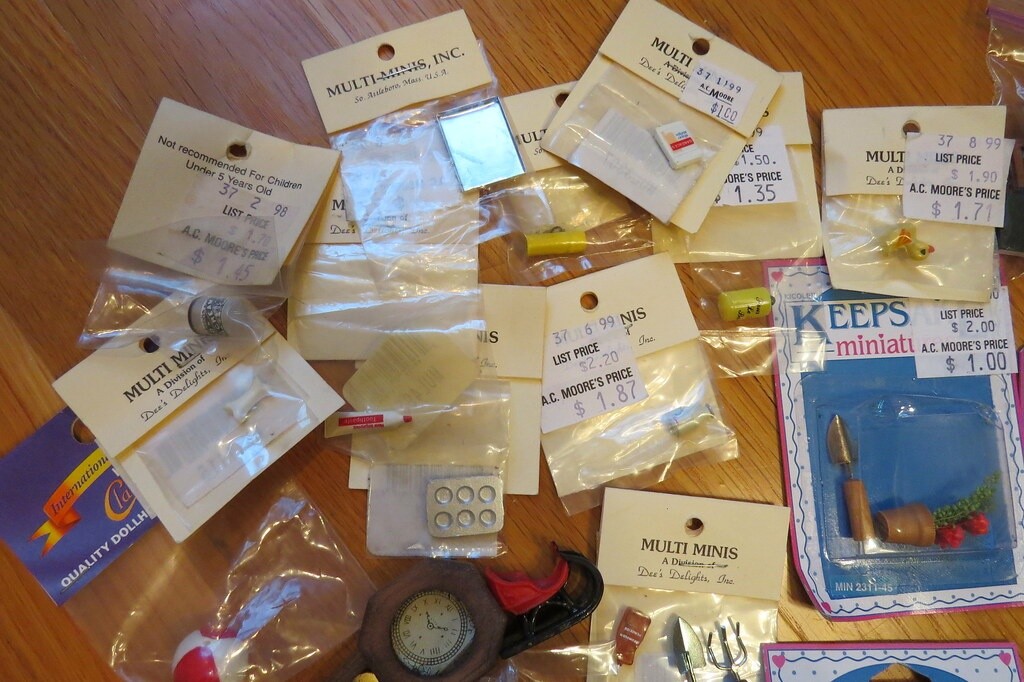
[355,558,506,681]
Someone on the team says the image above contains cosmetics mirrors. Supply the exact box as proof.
[434,95,526,192]
